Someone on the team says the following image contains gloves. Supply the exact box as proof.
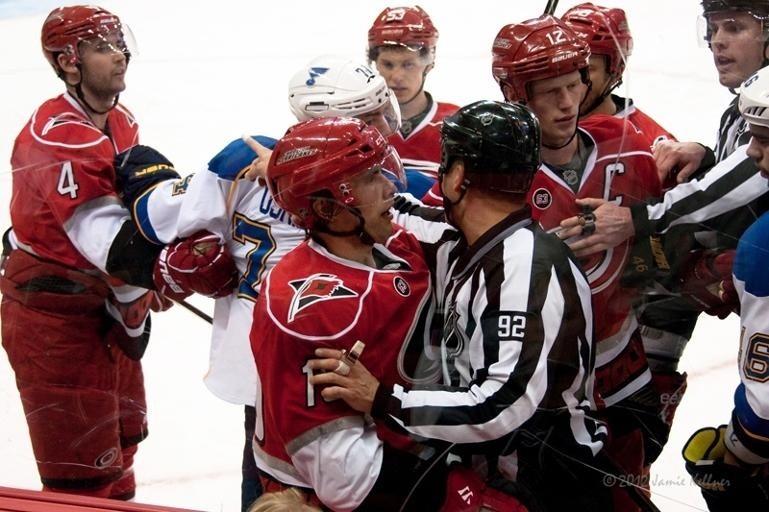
[682,425,769,512]
[152,235,239,302]
[440,462,529,512]
[114,145,182,209]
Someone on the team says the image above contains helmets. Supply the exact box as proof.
[697,0,769,61]
[438,99,541,194]
[267,116,408,229]
[492,14,593,103]
[367,5,438,67]
[561,2,634,81]
[41,4,139,78]
[738,66,769,127]
[287,55,402,139]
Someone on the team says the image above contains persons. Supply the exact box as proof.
[355,5,468,192]
[704,66,768,511]
[559,0,769,282]
[129,50,449,508]
[238,119,532,512]
[310,97,596,502]
[487,16,724,493]
[536,0,712,365]
[2,1,233,498]
